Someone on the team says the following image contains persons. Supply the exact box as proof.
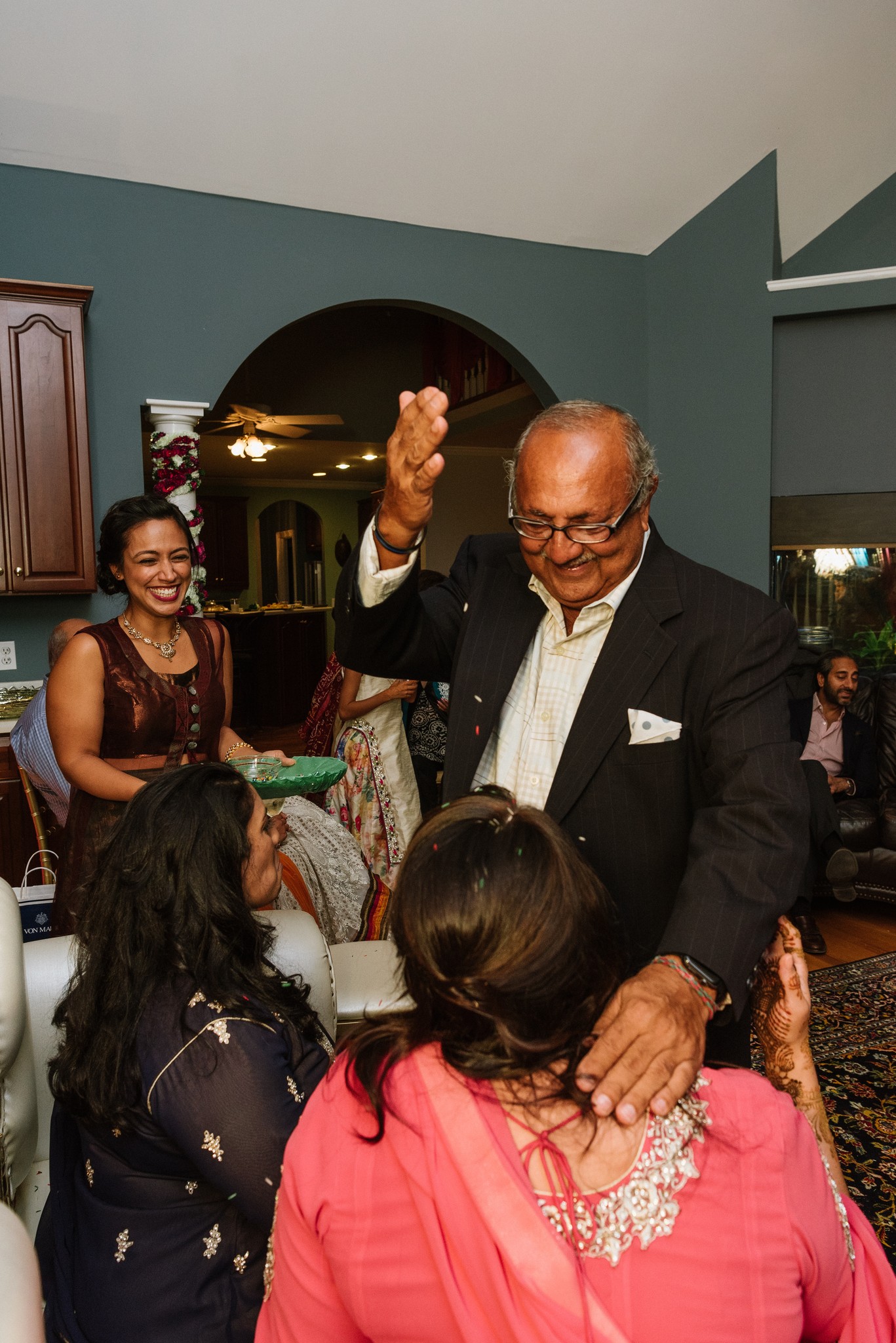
[350,388,821,1133]
[788,655,885,955]
[9,617,98,831]
[232,771,895,1343]
[48,512,297,944]
[32,752,351,1343]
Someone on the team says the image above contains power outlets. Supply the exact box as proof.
[0,638,16,672]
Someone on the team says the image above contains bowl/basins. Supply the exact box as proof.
[226,755,282,784]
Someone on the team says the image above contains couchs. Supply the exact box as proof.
[820,669,896,913]
[0,871,419,1342]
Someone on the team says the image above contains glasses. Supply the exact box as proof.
[508,478,644,543]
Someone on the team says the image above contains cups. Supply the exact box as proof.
[281,602,288,608]
[230,598,240,613]
[293,601,303,608]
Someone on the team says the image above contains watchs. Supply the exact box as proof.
[674,951,728,1005]
[844,777,852,794]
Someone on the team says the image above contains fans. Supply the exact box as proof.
[198,402,347,458]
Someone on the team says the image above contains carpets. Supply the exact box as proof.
[746,947,896,1283]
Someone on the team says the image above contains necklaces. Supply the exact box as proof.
[122,605,182,663]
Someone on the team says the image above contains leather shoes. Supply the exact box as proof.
[793,915,827,953]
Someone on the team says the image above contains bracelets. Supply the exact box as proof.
[649,955,719,1021]
[224,741,255,764]
[275,839,282,849]
[373,500,429,555]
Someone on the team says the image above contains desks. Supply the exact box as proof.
[201,605,327,729]
[0,718,59,888]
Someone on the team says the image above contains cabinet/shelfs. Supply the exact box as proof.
[0,277,104,602]
